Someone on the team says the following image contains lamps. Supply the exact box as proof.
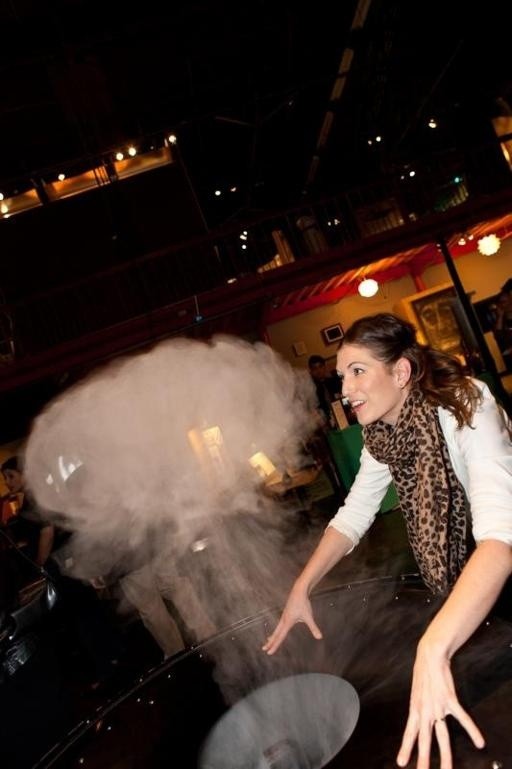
[249,450,337,521]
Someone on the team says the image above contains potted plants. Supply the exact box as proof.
[404,282,466,355]
[321,322,345,344]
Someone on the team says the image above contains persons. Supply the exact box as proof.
[1,454,89,570]
[420,296,462,351]
[305,354,341,412]
[493,276,512,346]
[73,485,218,666]
[259,311,512,769]
